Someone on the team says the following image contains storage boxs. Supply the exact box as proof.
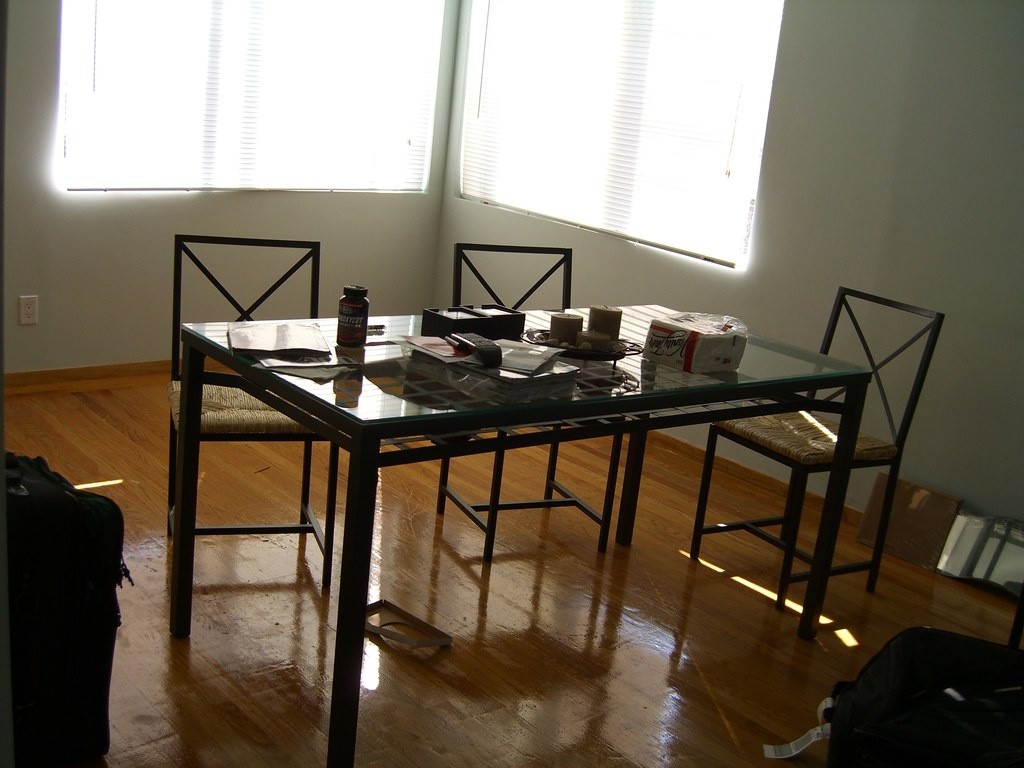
[460,304,526,344]
[421,305,493,342]
[850,472,962,577]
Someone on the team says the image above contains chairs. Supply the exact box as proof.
[165,234,338,590]
[435,242,626,565]
[686,285,946,612]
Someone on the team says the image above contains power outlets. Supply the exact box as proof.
[18,296,39,325]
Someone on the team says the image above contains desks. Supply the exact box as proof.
[163,301,879,768]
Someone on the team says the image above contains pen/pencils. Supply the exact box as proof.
[368,325,385,329]
[445,334,476,351]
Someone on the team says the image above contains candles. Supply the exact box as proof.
[577,329,610,352]
[550,312,583,345]
[589,304,623,344]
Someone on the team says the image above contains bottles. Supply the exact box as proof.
[337,284,370,348]
[333,345,365,408]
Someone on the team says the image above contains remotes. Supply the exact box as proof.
[452,333,502,368]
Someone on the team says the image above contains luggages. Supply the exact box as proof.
[822,625,1024,768]
[0,450,134,768]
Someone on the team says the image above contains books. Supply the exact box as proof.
[393,335,582,404]
